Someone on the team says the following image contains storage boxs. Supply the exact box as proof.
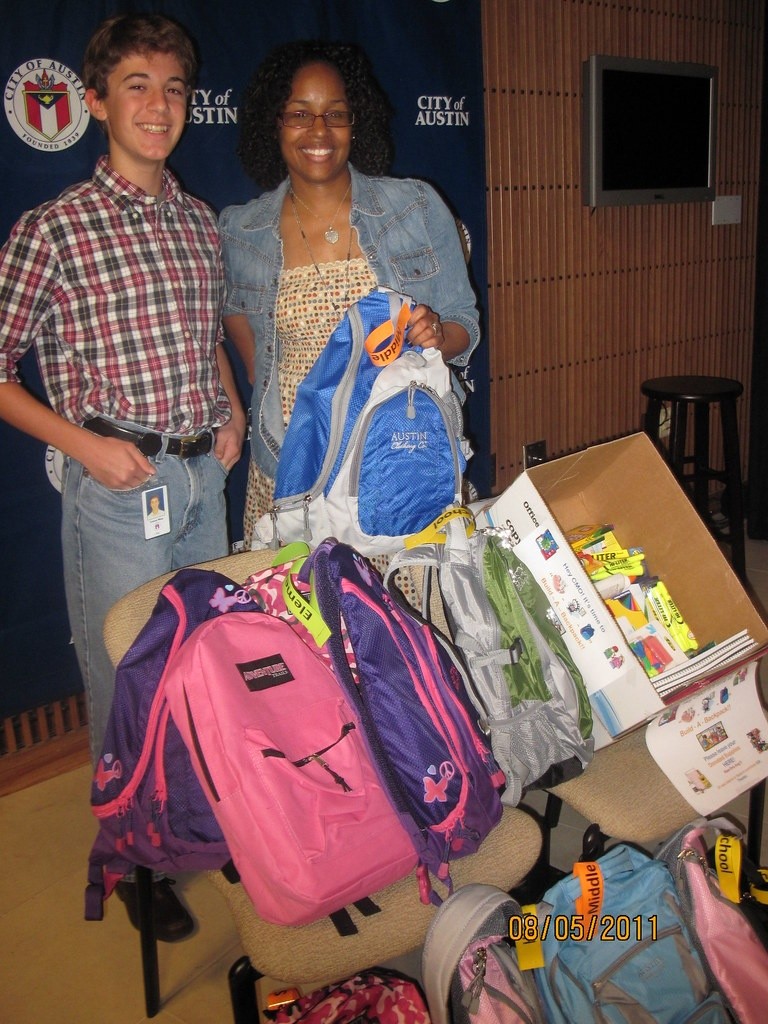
[477,430,768,755]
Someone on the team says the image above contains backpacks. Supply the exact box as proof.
[420,883,549,1024]
[263,966,431,1024]
[654,812,768,1024]
[249,286,475,557]
[515,823,731,1024]
[84,509,595,922]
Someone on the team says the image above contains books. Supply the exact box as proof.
[565,524,759,698]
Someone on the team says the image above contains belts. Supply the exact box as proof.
[82,416,220,459]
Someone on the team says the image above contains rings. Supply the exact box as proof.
[431,323,440,336]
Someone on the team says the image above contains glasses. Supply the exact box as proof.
[275,110,355,129]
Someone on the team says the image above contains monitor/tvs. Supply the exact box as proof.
[583,54,718,207]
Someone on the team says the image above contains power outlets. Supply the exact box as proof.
[659,407,671,439]
[522,439,547,470]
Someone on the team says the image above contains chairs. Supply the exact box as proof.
[103,547,545,1024]
[545,720,768,867]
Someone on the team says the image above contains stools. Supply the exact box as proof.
[640,376,746,587]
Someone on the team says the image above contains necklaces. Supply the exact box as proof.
[288,189,353,323]
[290,184,353,244]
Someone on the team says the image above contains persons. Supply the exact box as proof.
[0,11,245,941]
[149,493,164,519]
[214,37,480,543]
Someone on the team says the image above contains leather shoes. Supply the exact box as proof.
[114,877,194,942]
